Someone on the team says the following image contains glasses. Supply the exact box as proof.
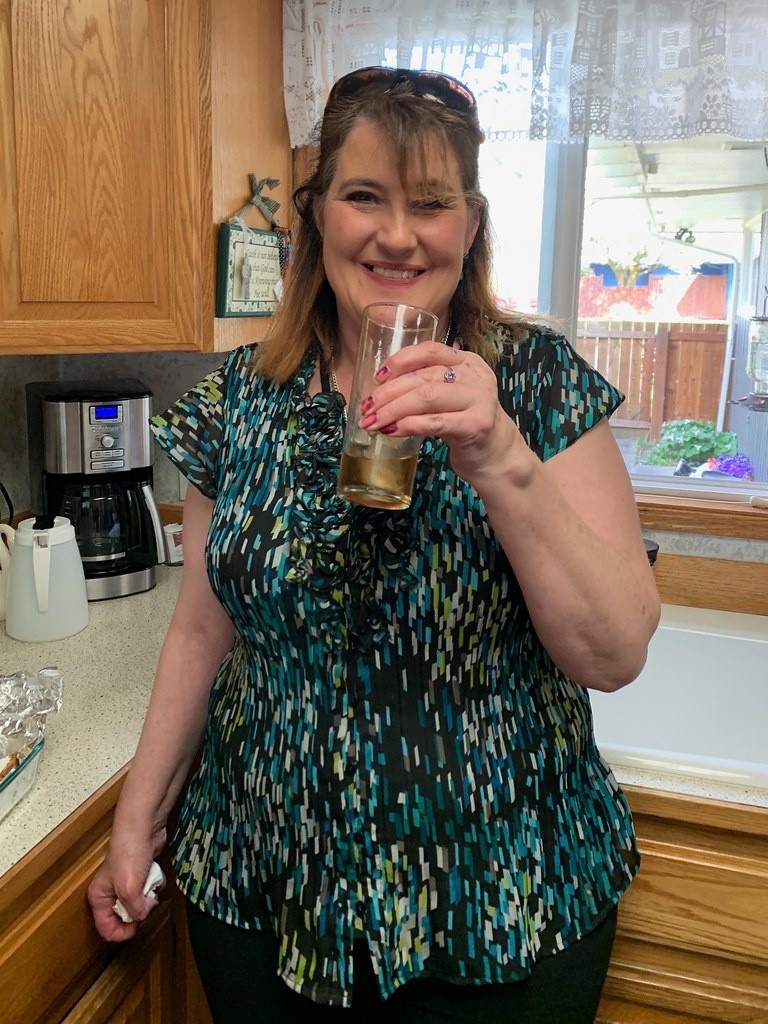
[331,65,475,118]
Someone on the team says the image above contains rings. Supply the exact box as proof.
[444,364,456,384]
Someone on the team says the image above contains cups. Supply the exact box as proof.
[337,303,440,511]
[643,538,659,568]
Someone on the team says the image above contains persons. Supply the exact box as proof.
[88,66,662,1024]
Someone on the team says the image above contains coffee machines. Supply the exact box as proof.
[24,378,168,603]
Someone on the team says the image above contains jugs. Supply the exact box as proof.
[6,515,89,643]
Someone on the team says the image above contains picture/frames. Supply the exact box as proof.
[216,223,287,318]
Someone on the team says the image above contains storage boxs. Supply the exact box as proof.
[0,737,46,825]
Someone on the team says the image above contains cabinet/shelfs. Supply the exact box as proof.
[0,750,213,1024]
[593,780,768,1024]
[0,0,287,356]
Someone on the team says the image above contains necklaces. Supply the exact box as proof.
[329,321,452,426]
